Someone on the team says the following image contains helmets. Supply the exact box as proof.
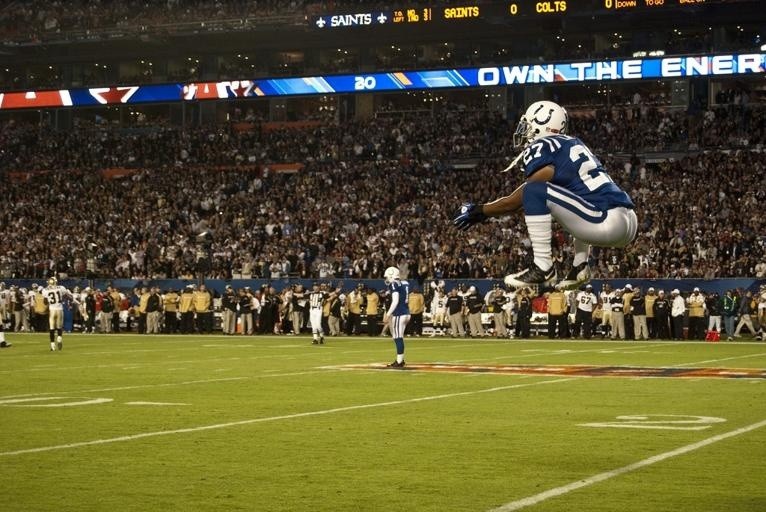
[383,266,400,285]
[511,100,566,149]
[49,277,57,285]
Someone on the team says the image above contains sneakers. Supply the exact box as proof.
[555,261,592,291]
[311,337,324,344]
[0,342,12,348]
[387,360,405,367]
[503,262,557,288]
[50,339,62,352]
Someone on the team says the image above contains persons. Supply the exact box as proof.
[378,265,411,367]
[309,281,329,345]
[40,276,81,351]
[0,0,766,348]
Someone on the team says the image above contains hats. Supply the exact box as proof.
[614,284,699,293]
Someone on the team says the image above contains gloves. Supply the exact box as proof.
[453,201,484,232]
